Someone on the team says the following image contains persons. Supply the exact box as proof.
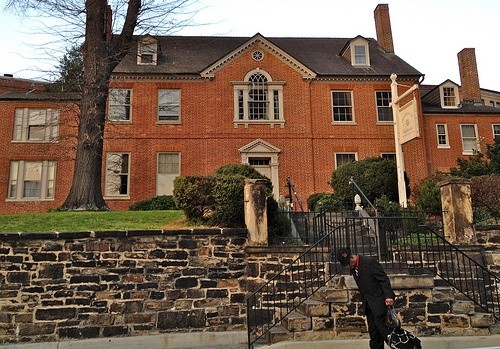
[337,245,422,349]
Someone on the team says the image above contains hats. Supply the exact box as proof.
[337,247,352,266]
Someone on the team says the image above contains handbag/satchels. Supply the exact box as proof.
[385,306,422,349]
